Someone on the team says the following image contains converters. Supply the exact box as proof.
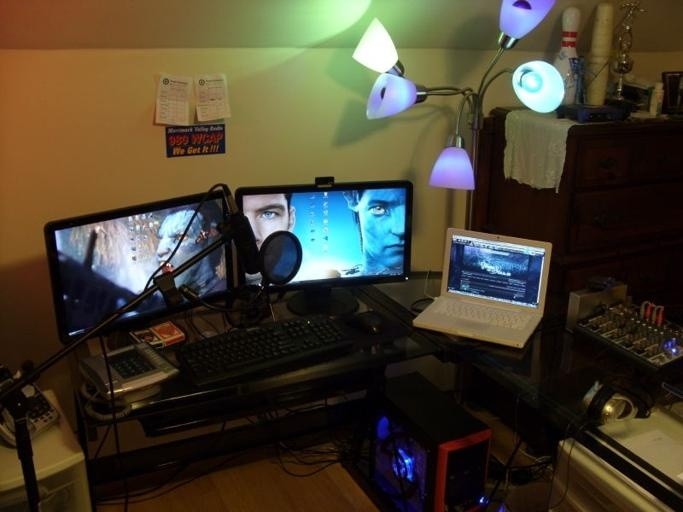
[510,468,531,485]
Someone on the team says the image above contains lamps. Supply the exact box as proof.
[352,18,405,77]
[511,60,565,113]
[366,73,428,120]
[497,0,555,51]
[429,134,476,190]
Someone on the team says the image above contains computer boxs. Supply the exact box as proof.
[367,372,493,512]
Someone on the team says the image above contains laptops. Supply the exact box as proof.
[411,228,552,349]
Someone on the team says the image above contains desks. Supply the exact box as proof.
[72,283,440,487]
[354,266,664,431]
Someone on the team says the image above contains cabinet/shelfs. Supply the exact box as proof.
[473,105,683,377]
[1,384,95,512]
[549,402,683,512]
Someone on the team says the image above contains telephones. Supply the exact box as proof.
[76,341,182,410]
[0,370,61,446]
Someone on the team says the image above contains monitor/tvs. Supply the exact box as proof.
[43,186,238,351]
[235,177,413,316]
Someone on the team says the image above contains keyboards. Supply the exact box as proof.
[172,312,357,391]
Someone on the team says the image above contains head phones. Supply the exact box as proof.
[577,378,639,425]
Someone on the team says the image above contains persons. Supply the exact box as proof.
[340,189,406,277]
[155,202,228,298]
[241,192,341,286]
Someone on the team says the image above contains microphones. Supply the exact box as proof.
[221,184,264,275]
